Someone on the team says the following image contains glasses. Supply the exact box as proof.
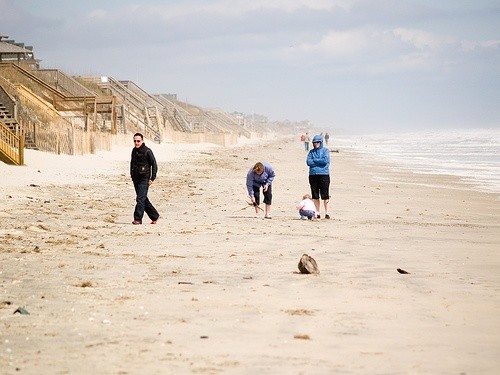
[314,141,320,143]
[134,140,142,142]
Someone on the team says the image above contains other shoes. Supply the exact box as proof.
[150,220,156,224]
[316,213,320,218]
[325,213,330,219]
[132,220,142,224]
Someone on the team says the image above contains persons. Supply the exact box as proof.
[301,132,329,151]
[296,194,316,221]
[245,162,276,219]
[129,132,160,225]
[306,134,331,219]
